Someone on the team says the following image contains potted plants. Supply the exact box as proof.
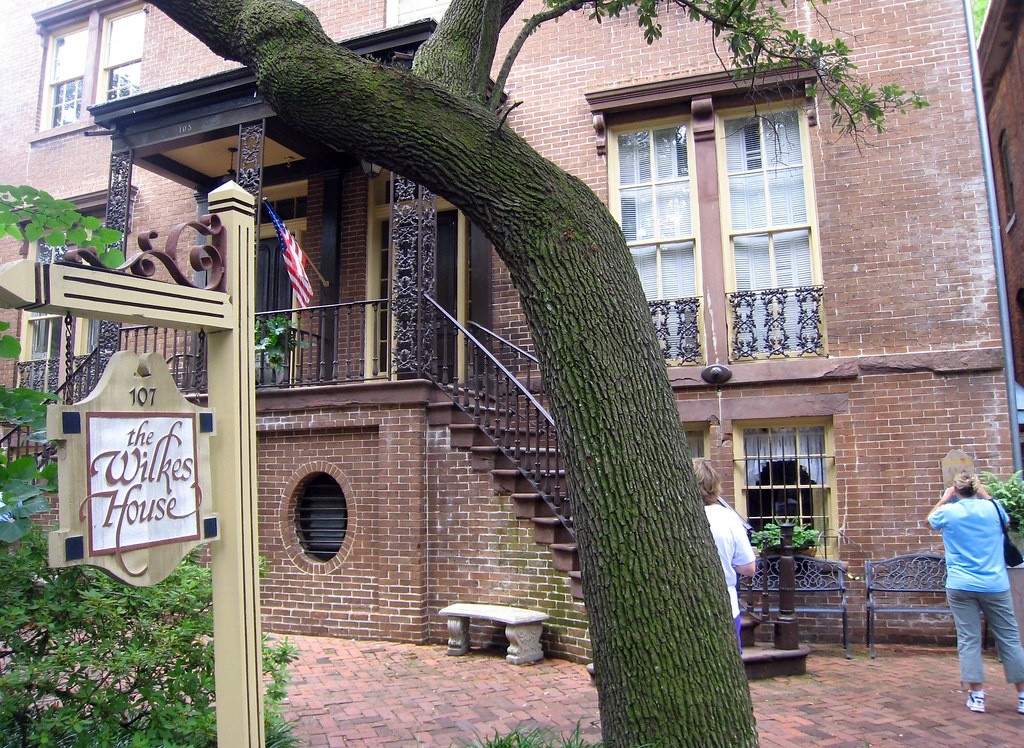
[254,317,320,388]
[751,523,824,557]
[979,470,1024,547]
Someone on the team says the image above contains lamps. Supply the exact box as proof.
[361,159,383,178]
[221,148,238,182]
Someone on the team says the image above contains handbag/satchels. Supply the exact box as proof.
[989,538,1024,567]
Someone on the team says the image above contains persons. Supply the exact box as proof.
[691,457,756,655]
[924,469,1024,713]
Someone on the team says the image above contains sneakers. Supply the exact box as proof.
[1017,697,1024,713]
[966,693,985,712]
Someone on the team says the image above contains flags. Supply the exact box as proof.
[264,197,313,317]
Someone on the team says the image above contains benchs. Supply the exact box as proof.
[735,553,852,659]
[438,603,549,665]
[864,554,1003,663]
